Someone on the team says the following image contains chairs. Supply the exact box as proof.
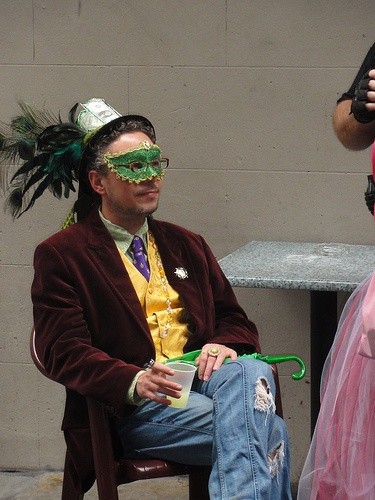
[30,325,211,500]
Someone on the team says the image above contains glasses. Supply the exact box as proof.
[114,158,169,173]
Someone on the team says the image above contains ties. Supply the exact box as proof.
[130,236,150,284]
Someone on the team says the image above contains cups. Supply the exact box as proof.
[163,362,197,409]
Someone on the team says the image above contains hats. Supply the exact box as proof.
[0,97,156,223]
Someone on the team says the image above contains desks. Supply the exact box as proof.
[217,242,375,440]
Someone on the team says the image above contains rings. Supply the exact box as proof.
[208,347,220,356]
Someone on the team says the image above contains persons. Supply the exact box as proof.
[30,98,292,500]
[297,43,375,500]
